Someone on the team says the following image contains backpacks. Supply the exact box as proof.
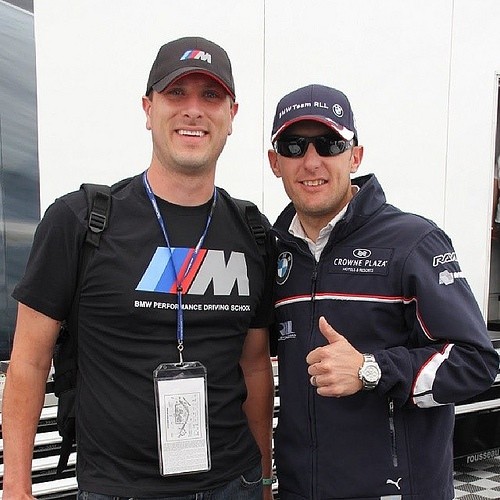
[49,183,266,444]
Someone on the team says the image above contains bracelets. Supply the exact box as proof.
[262,478,277,486]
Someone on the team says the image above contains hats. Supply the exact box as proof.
[270,84,358,149]
[146,37,235,102]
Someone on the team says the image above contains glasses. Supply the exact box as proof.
[277,133,355,158]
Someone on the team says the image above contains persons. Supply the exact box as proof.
[265,84,499,499]
[0,36,282,500]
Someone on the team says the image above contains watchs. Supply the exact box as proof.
[360,351,382,393]
